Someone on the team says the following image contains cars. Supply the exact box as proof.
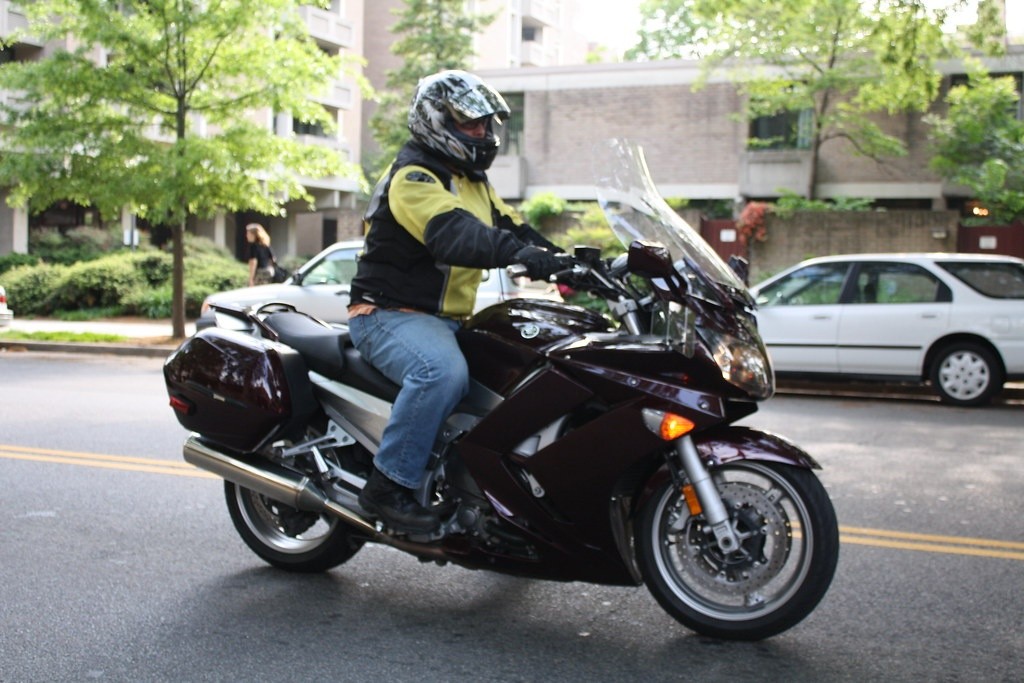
[195,240,562,358]
[737,252,1022,409]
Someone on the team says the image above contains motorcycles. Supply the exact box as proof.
[162,136,840,642]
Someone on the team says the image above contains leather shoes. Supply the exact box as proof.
[357,469,440,533]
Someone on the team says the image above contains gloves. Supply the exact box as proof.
[507,246,562,284]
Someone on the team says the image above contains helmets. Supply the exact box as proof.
[407,70,510,171]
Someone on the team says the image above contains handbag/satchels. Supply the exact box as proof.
[267,263,293,283]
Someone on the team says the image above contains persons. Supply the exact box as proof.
[346,70,566,533]
[246,223,275,287]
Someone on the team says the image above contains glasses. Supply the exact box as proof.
[452,115,490,132]
